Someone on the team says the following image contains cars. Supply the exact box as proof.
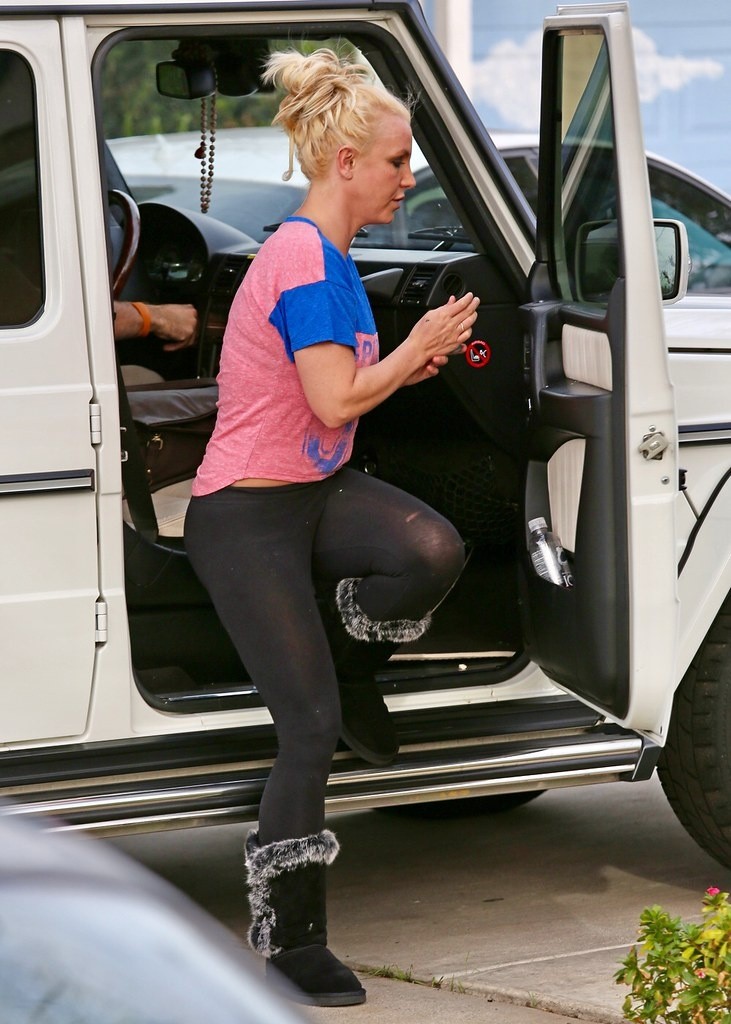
[1,124,731,296]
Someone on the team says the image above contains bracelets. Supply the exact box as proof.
[132,302,150,337]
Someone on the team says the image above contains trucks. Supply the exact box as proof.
[0,0,731,872]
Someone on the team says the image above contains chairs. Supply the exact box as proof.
[408,199,462,233]
[116,381,377,604]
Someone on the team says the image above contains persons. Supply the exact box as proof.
[184,50,479,1006]
[113,301,197,352]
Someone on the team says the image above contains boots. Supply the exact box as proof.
[323,576,431,767]
[244,828,366,1005]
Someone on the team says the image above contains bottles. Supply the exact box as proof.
[527,517,576,590]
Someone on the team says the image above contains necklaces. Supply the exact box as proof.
[199,71,215,213]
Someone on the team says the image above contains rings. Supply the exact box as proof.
[460,323,465,330]
